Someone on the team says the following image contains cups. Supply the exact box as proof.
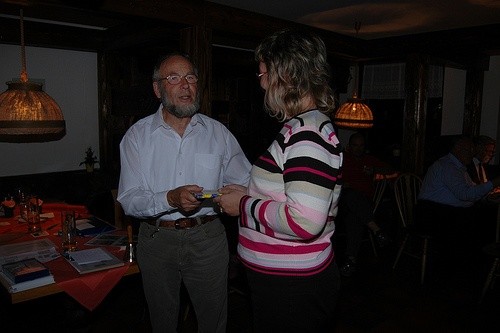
[61,210,77,250]
[18,193,28,218]
[26,197,40,226]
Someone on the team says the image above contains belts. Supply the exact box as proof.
[143,214,222,230]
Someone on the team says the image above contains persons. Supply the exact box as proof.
[117,54,253,333]
[343,133,392,276]
[212,28,343,333]
[416,134,500,229]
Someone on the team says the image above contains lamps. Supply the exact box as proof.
[0,0,67,141]
[334,23,375,128]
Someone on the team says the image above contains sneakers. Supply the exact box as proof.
[371,229,393,247]
[341,259,360,277]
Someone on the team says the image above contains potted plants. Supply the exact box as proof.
[78,146,100,172]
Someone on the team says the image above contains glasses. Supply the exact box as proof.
[255,72,266,80]
[159,74,200,85]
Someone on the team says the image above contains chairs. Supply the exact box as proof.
[391,172,437,282]
[477,212,500,306]
[111,189,139,232]
[350,169,388,256]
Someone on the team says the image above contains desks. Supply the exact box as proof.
[59,170,117,211]
[0,201,141,333]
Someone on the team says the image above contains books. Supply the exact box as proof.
[66,247,124,273]
[0,237,62,294]
